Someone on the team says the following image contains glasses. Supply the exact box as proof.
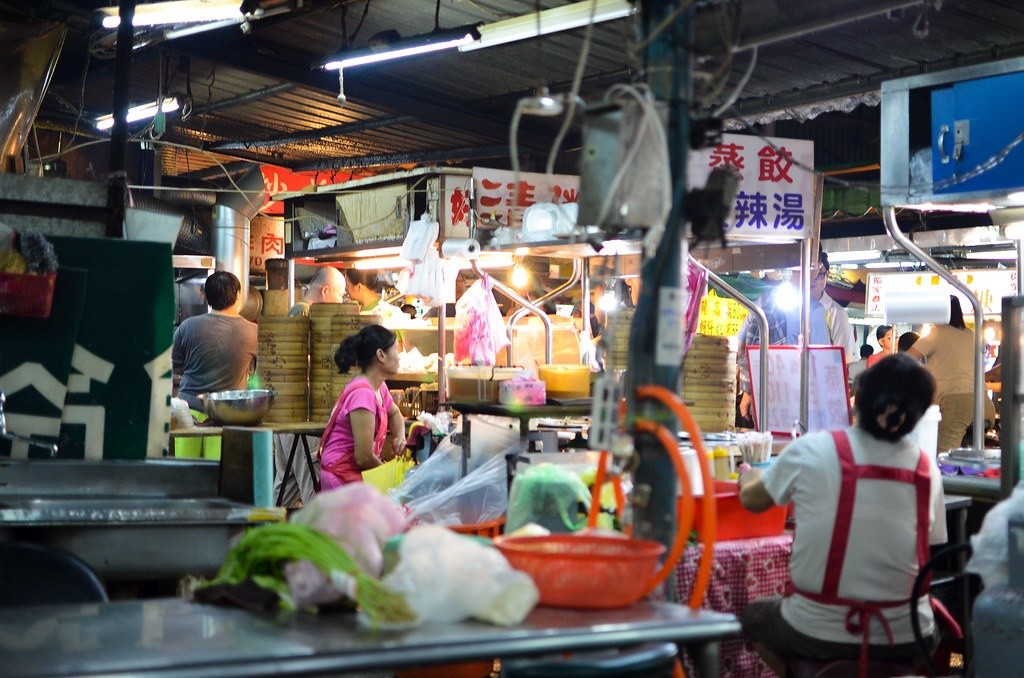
[815,270,828,280]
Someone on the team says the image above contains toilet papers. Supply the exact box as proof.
[442,237,481,260]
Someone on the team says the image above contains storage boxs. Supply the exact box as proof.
[677,479,786,542]
[445,365,525,404]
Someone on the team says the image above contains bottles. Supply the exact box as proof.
[714,446,729,481]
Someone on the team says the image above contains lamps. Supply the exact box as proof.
[953,247,1017,260]
[519,0,564,116]
[92,92,184,131]
[311,0,640,112]
[95,0,264,34]
[353,249,515,270]
[775,267,799,310]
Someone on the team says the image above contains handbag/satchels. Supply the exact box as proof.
[361,452,418,494]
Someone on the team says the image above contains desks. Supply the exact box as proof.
[677,527,793,678]
[451,400,592,476]
[945,494,972,545]
[0,596,742,678]
[169,422,326,506]
[943,474,1001,498]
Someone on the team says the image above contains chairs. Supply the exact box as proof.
[910,543,974,678]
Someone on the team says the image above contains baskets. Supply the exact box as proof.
[492,534,667,608]
[0,269,57,317]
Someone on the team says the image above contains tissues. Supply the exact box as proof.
[497,369,547,406]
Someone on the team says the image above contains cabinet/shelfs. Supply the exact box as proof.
[880,57,1024,207]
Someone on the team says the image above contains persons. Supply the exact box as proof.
[401,304,417,319]
[273,266,347,508]
[737,240,1001,454]
[171,270,258,414]
[738,353,948,678]
[317,324,408,493]
[506,268,624,371]
[345,268,403,321]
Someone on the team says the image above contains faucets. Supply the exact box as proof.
[0,388,59,458]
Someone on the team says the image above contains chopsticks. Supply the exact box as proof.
[738,430,773,464]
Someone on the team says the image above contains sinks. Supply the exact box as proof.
[0,495,262,575]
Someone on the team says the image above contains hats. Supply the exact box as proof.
[856,353,938,442]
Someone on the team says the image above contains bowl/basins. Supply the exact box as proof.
[196,389,278,427]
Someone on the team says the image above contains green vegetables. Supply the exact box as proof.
[197,523,418,623]
